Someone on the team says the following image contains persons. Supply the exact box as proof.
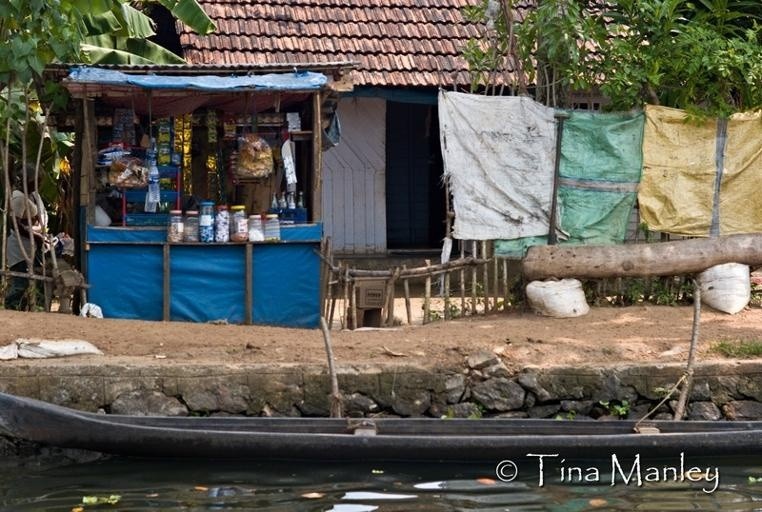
[4,162,50,311]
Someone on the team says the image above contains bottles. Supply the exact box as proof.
[147,157,162,206]
[166,201,281,241]
[270,190,307,210]
[145,136,159,175]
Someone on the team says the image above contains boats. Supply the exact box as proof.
[0,390,761,472]
